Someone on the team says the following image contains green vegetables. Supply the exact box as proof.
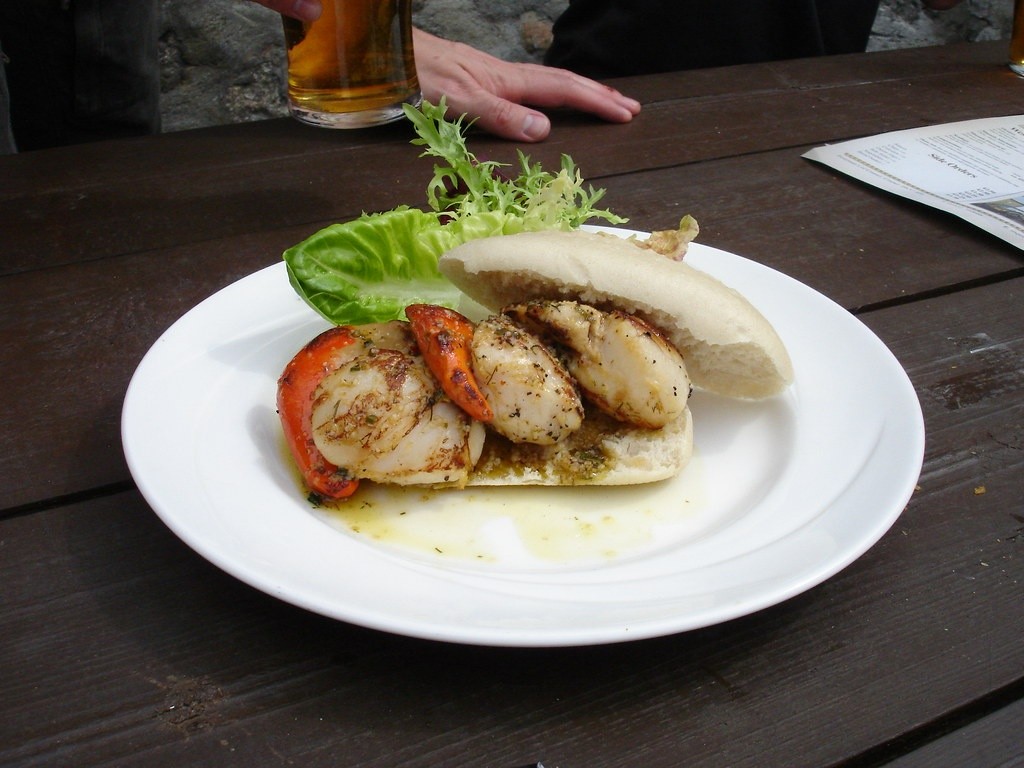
[279,96,630,326]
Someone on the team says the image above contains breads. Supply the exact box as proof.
[434,229,796,399]
[457,397,692,486]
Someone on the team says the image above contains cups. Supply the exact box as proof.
[1007,0,1024,78]
[282,0,423,128]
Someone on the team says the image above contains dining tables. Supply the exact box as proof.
[0,39,1024,768]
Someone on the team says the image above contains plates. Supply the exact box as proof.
[120,225,926,647]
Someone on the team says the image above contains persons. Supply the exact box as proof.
[545,0,963,81]
[0,1,642,155]
